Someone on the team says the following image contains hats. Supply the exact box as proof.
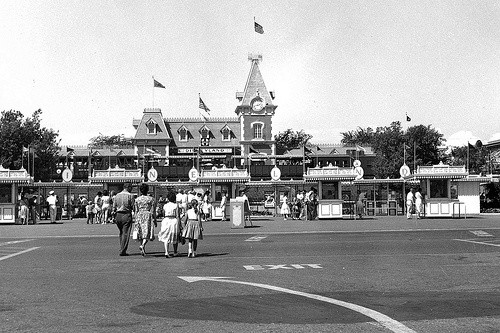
[49,190,54,194]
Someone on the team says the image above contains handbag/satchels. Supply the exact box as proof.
[132,230,140,241]
[179,229,186,245]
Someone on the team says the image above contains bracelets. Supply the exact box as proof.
[153,220,157,222]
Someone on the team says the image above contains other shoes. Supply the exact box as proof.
[165,252,179,257]
[187,249,196,257]
[139,245,146,256]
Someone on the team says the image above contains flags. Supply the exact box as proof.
[154,79,165,88]
[200,98,210,115]
[407,116,411,121]
[254,22,264,35]
[67,147,74,152]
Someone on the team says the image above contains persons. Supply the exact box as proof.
[157,191,183,258]
[260,195,274,216]
[406,184,423,220]
[73,190,117,224]
[480,192,485,210]
[19,190,62,226]
[451,185,456,195]
[113,181,134,256]
[182,200,204,257]
[149,186,211,222]
[217,191,227,222]
[327,189,334,199]
[354,189,364,219]
[241,192,254,228]
[133,183,157,257]
[280,186,319,221]
